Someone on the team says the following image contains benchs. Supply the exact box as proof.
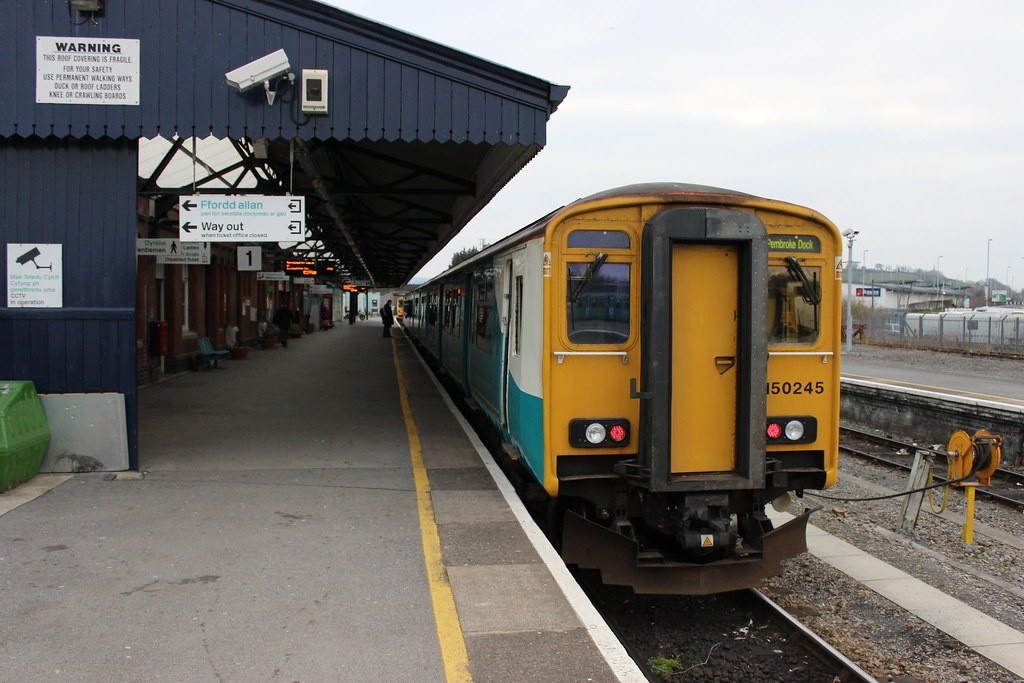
[196,337,230,369]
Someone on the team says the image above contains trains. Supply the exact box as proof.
[400,185,841,594]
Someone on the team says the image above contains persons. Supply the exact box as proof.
[349,301,357,324]
[320,302,329,330]
[380,300,394,338]
[271,301,294,347]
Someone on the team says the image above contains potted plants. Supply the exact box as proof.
[256,330,278,350]
[304,323,314,334]
[288,324,303,339]
[227,339,251,360]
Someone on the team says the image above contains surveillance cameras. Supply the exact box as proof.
[842,230,851,236]
[851,228,859,235]
[225,48,290,94]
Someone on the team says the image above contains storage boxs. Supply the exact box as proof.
[0,380,50,495]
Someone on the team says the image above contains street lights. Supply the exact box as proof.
[1006,266,1011,291]
[984,238,993,306]
[861,249,868,304]
[841,228,859,353]
[937,255,942,300]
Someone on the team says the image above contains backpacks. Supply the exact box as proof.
[380,309,386,319]
